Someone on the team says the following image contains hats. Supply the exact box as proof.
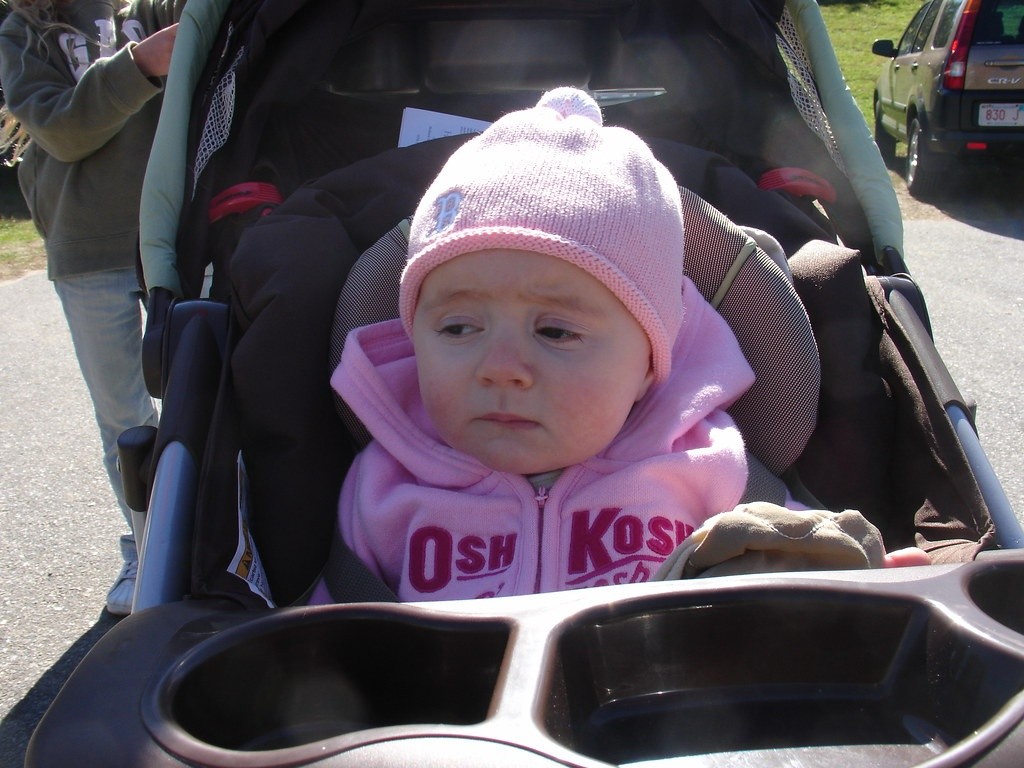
[397,86,685,385]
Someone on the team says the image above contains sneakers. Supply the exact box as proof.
[107,561,140,616]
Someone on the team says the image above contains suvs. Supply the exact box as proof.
[871,0,1024,197]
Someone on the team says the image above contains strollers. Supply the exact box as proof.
[23,0,1024,768]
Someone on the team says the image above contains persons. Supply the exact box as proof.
[0,0,186,611]
[302,85,936,604]
